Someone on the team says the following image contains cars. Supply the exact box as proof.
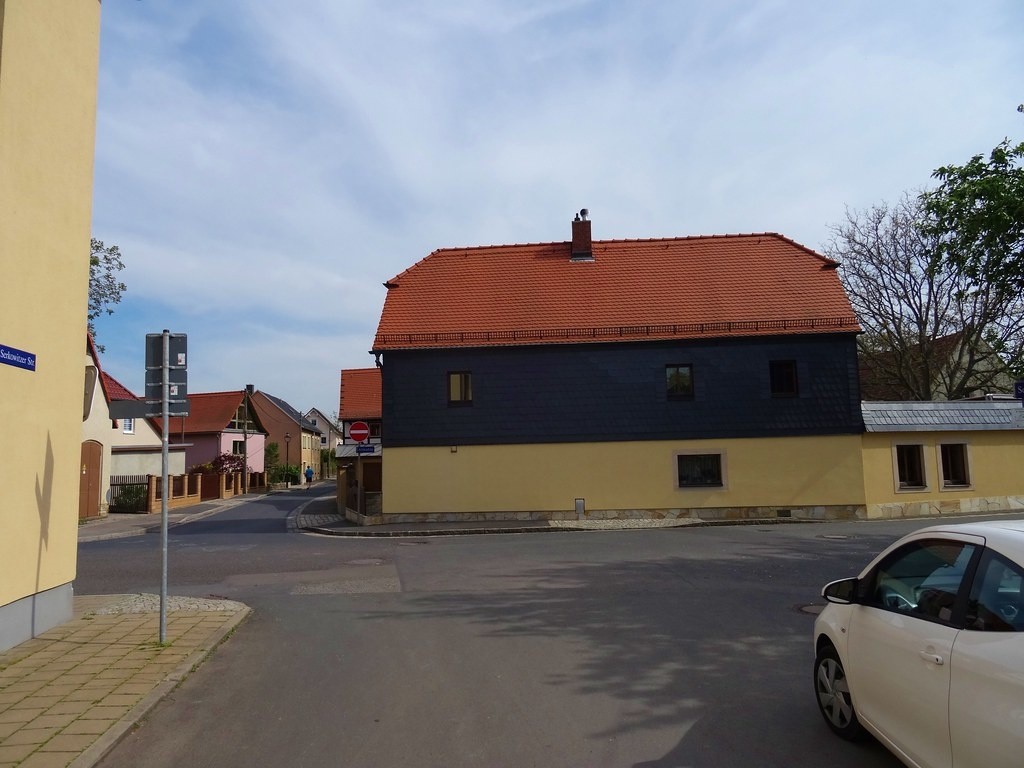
[813,520,1023,768]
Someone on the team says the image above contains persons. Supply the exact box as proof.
[303,465,314,491]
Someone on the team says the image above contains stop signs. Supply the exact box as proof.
[349,422,369,442]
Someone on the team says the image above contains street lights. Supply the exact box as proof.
[284,432,292,489]
